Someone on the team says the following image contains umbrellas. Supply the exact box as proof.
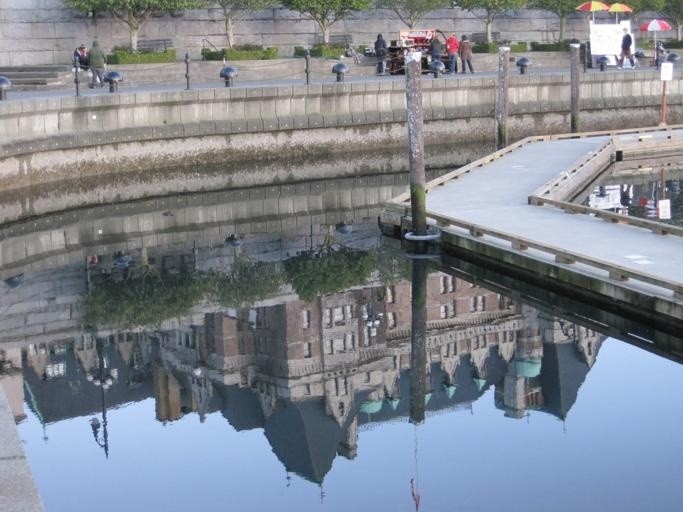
[574,0,608,22]
[634,7,671,18]
[602,1,632,23]
[638,19,671,58]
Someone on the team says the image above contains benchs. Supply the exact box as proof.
[137,36,173,52]
[470,32,500,43]
[317,34,352,50]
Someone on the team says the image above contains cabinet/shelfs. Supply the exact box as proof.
[385,43,455,76]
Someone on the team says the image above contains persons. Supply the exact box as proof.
[457,34,474,74]
[618,183,633,207]
[374,32,386,72]
[616,27,634,69]
[73,43,88,68]
[638,183,650,198]
[85,39,107,88]
[445,32,459,74]
[428,35,442,73]
[636,195,649,208]
